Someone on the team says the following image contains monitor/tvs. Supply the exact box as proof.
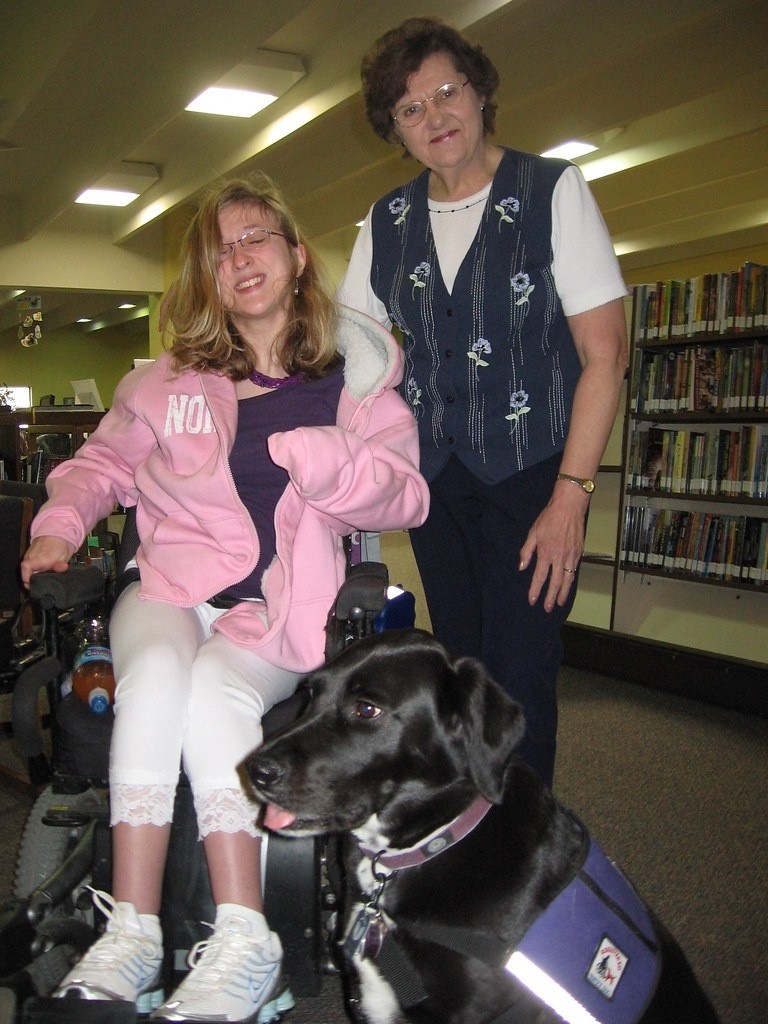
[71,378,105,413]
[0,386,33,409]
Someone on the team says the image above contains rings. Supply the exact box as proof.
[564,568,576,573]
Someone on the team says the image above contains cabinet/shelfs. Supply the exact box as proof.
[568,285,767,669]
[0,404,109,555]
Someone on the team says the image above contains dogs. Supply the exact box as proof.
[239,626,724,1024]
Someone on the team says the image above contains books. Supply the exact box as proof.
[616,262,768,592]
[17,405,94,412]
[0,446,122,598]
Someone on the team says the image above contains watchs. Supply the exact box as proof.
[557,474,595,494]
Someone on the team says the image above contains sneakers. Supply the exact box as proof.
[51,885,164,1013]
[149,915,296,1024]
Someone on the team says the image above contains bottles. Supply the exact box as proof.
[73,617,116,714]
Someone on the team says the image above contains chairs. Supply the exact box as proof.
[1,495,56,796]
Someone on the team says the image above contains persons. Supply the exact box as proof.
[19,179,430,1023]
[337,19,631,800]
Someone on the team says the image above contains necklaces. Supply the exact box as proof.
[428,197,488,214]
[244,368,309,388]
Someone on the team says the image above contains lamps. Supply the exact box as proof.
[74,160,159,209]
[185,47,306,118]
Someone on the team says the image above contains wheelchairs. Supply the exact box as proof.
[0,437,422,1024]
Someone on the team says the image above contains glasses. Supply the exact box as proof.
[218,230,286,262]
[392,79,470,128]
